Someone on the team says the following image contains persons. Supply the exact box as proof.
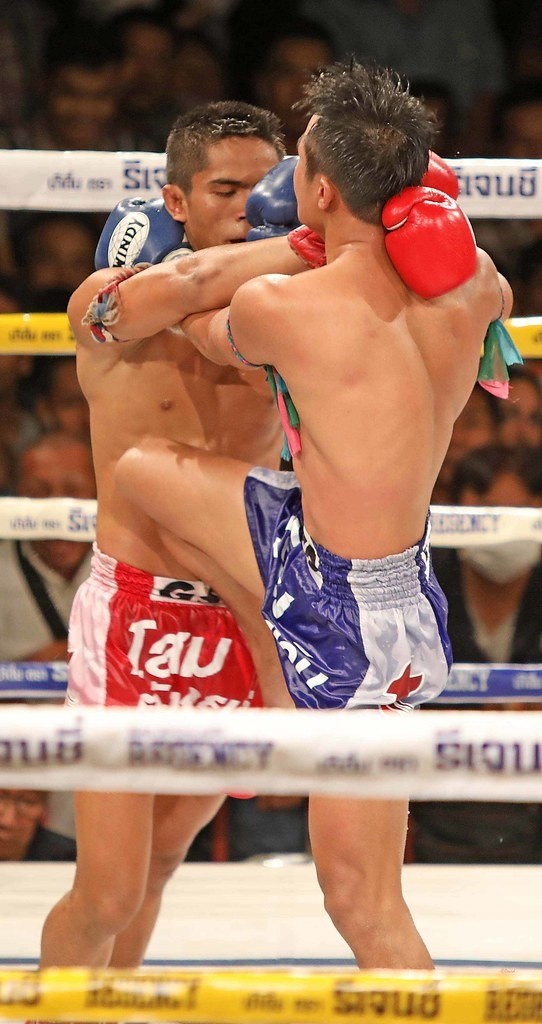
[96,52,513,969]
[0,0,542,865]
[42,101,480,968]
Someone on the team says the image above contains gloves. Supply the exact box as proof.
[381,185,479,299]
[287,150,460,270]
[245,157,303,243]
[95,197,194,271]
